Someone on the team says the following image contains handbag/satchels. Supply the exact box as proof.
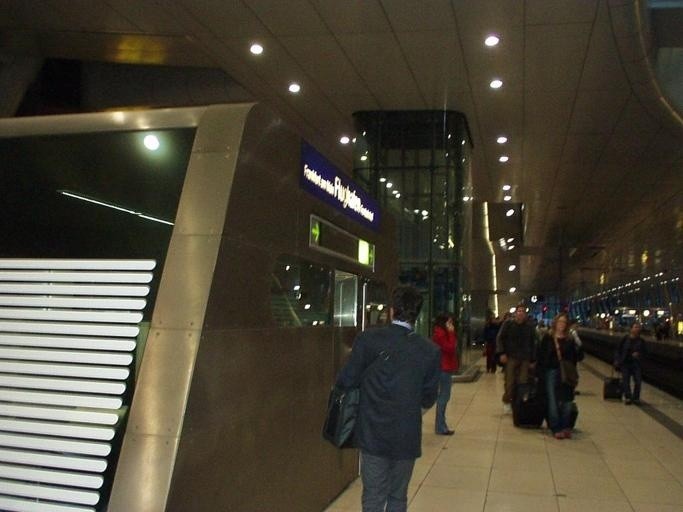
[560,360,577,388]
[323,388,362,450]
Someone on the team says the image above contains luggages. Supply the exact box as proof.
[512,380,546,429]
[603,368,625,402]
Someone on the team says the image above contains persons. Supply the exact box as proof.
[480,302,585,442]
[334,282,442,511]
[613,323,652,408]
[603,313,670,340]
[431,311,460,434]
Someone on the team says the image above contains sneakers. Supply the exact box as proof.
[442,430,454,435]
[625,400,642,406]
[554,430,571,440]
